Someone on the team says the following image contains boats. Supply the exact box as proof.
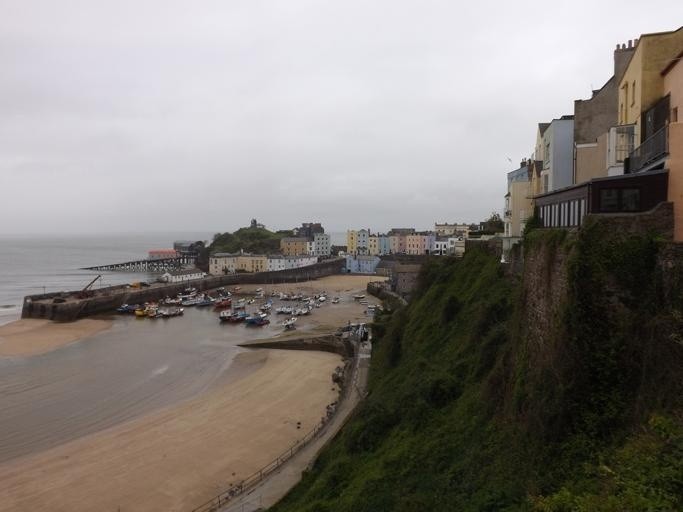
[115,281,343,330]
[351,293,380,312]
